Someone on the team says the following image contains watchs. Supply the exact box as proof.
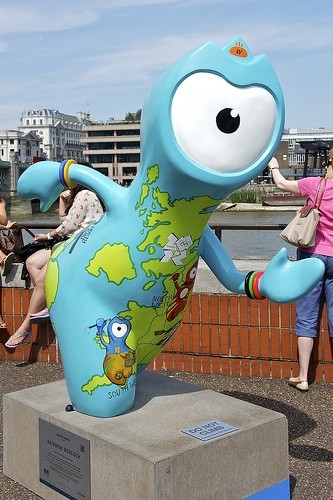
[272,166,278,169]
[47,232,54,240]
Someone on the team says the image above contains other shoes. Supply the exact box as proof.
[289,377,308,390]
[1,252,15,276]
[0,319,7,329]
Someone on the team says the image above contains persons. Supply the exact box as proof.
[269,148,333,390]
[0,162,105,349]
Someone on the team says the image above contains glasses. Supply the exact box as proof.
[327,160,333,168]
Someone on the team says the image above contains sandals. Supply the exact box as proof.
[29,307,50,323]
[4,326,32,348]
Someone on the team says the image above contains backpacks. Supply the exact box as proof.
[0,223,36,256]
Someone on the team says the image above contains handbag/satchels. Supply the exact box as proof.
[15,236,70,261]
[279,179,327,248]
